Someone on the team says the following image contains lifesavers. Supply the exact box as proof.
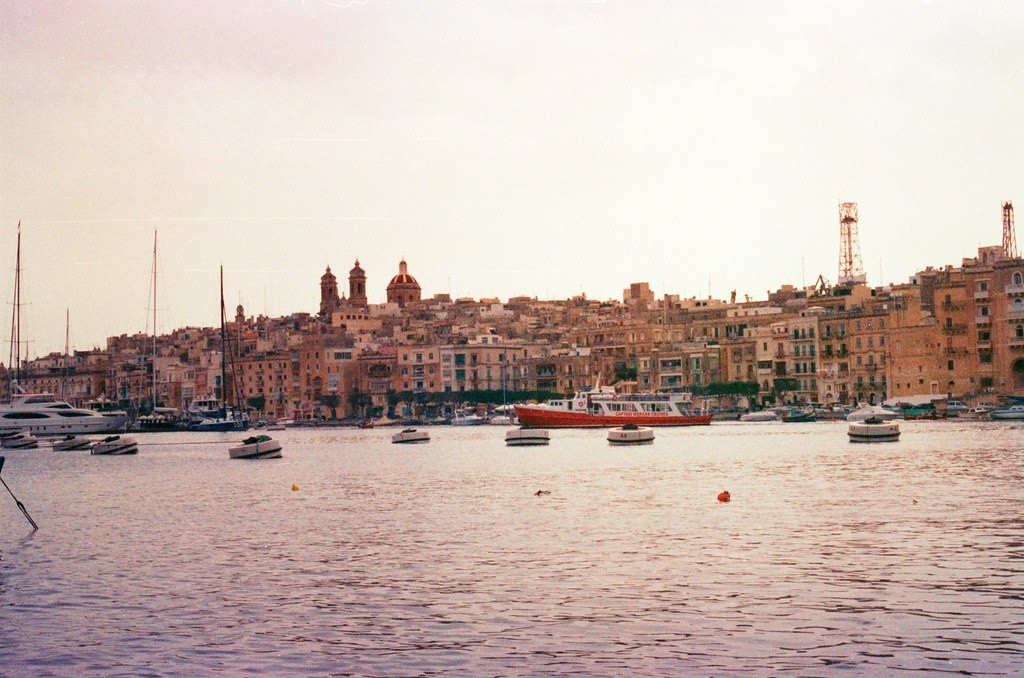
[579,402,583,407]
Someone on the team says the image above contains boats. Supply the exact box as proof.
[491,416,521,425]
[451,410,484,425]
[356,422,375,428]
[780,409,816,422]
[266,424,285,430]
[1,402,128,436]
[848,404,896,420]
[740,411,777,421]
[905,415,939,420]
[990,406,1024,419]
[815,407,847,419]
[430,420,452,425]
[514,370,712,428]
[276,418,295,427]
[957,406,990,418]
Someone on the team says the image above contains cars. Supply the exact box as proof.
[893,402,914,415]
[977,401,995,410]
[909,403,936,415]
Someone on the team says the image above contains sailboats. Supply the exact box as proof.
[119,229,206,431]
[179,264,251,432]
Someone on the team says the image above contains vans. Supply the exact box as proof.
[947,401,969,410]
